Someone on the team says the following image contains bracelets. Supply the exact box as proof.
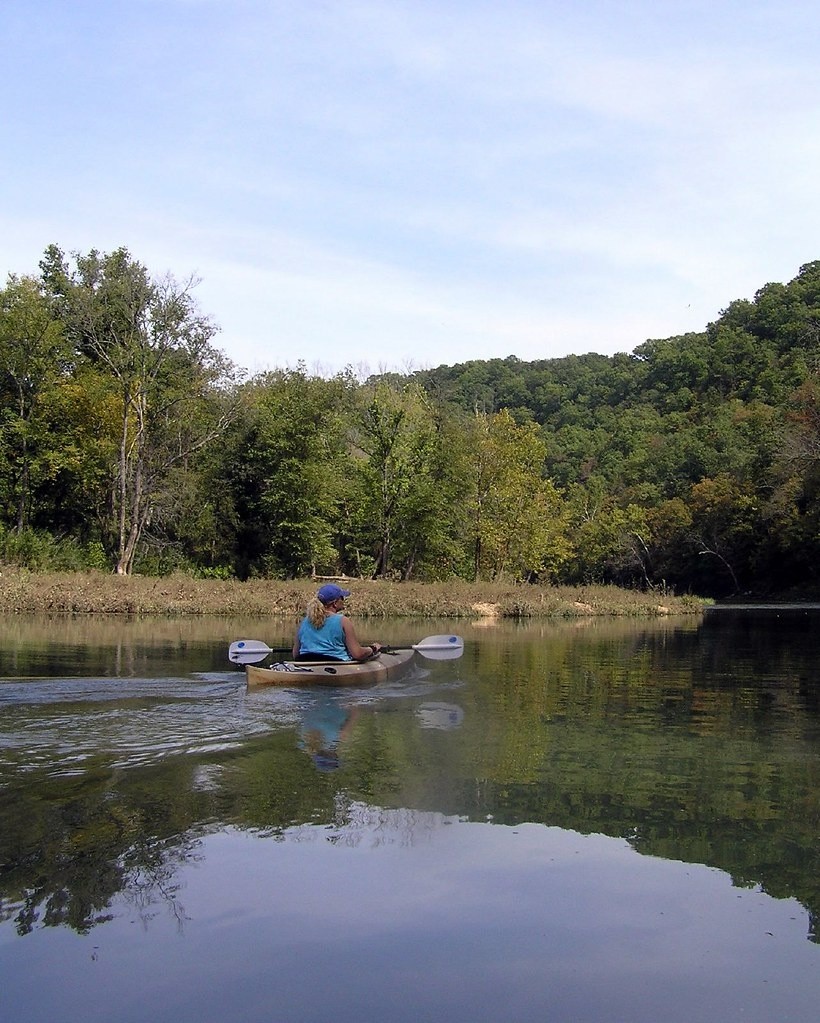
[369,646,377,655]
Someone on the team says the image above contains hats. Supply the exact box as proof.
[318,585,351,603]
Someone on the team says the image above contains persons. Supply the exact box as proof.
[293,584,381,660]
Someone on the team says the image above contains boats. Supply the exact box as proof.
[246,649,418,687]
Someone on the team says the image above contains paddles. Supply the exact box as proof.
[228,634,465,664]
